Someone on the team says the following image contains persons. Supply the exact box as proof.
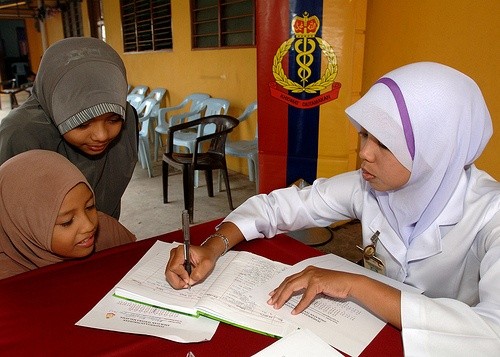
[0,37,139,279]
[164,62,500,357]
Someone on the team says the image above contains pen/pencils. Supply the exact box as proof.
[181,209,191,291]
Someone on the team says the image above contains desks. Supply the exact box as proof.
[0,216,404,357]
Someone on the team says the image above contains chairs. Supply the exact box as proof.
[126,84,258,225]
[11,62,29,87]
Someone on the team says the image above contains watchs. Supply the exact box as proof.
[200,233,229,255]
